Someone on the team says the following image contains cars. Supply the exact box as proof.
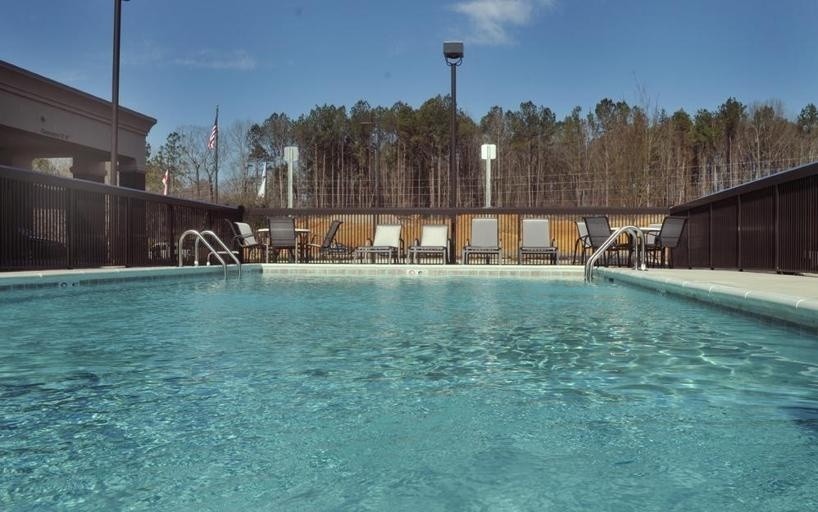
[0,224,65,268]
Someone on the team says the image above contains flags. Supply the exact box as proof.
[208,116,217,151]
[161,168,172,195]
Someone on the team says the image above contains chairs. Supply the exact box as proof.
[224,217,342,264]
[408,224,451,265]
[573,216,690,268]
[355,224,401,265]
[462,218,501,264]
[520,219,558,265]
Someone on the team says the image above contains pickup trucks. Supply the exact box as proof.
[146,241,193,264]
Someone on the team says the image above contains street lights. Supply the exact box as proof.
[441,38,465,205]
